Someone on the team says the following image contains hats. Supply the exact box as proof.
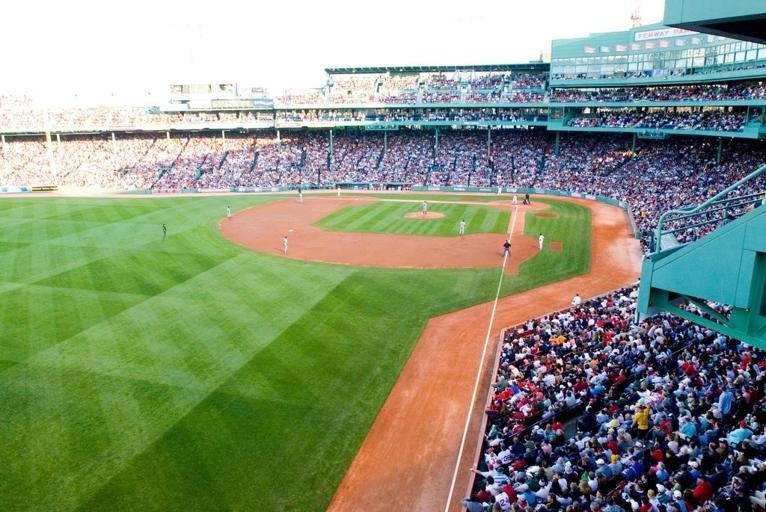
[487,366,747,486]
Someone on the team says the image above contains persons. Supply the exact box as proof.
[459,278,766,512]
[0,72,765,258]
